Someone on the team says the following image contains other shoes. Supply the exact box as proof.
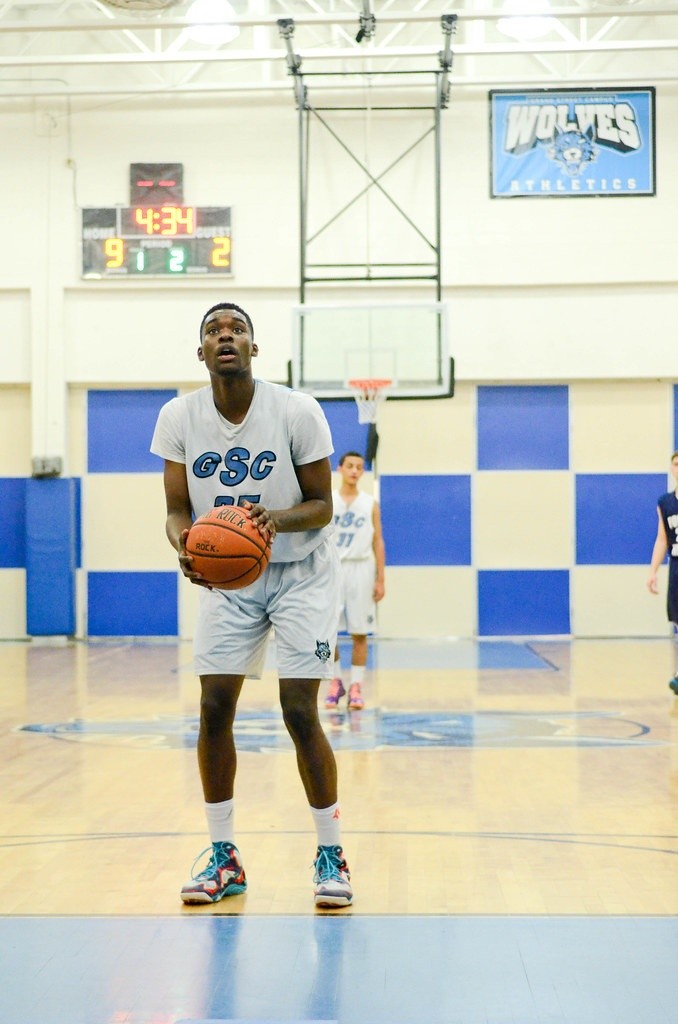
[348,683,364,709]
[670,672,678,695]
[324,679,345,709]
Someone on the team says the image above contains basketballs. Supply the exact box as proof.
[183,505,271,591]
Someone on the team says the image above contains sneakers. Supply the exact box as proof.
[180,841,247,903]
[312,845,353,907]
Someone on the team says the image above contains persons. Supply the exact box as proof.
[152,304,354,908]
[323,452,386,709]
[649,451,678,692]
